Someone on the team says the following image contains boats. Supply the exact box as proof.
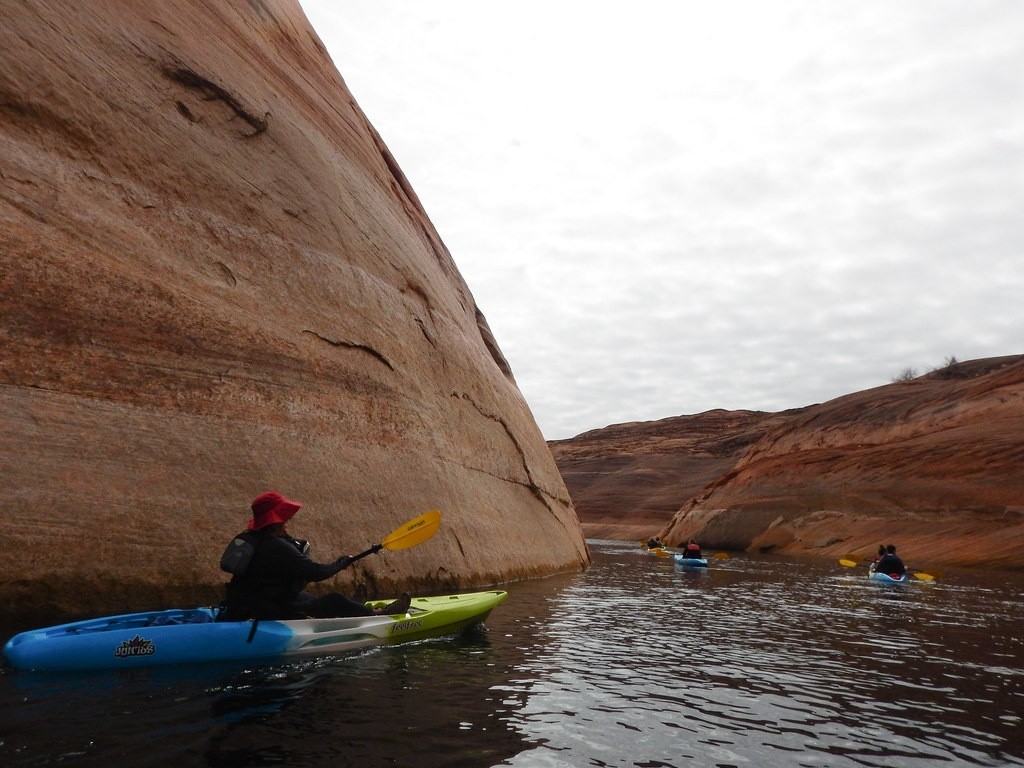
[5,589,513,676]
[645,546,668,557]
[869,568,907,582]
[673,552,709,566]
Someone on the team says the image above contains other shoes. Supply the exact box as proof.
[351,583,368,607]
[379,592,410,616]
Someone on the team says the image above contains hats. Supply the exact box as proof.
[246,491,303,531]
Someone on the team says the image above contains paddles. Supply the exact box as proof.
[313,510,441,580]
[656,551,730,560]
[839,559,934,581]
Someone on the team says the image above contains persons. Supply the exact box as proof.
[215,491,412,623]
[682,538,702,559]
[870,543,905,579]
[647,537,664,549]
